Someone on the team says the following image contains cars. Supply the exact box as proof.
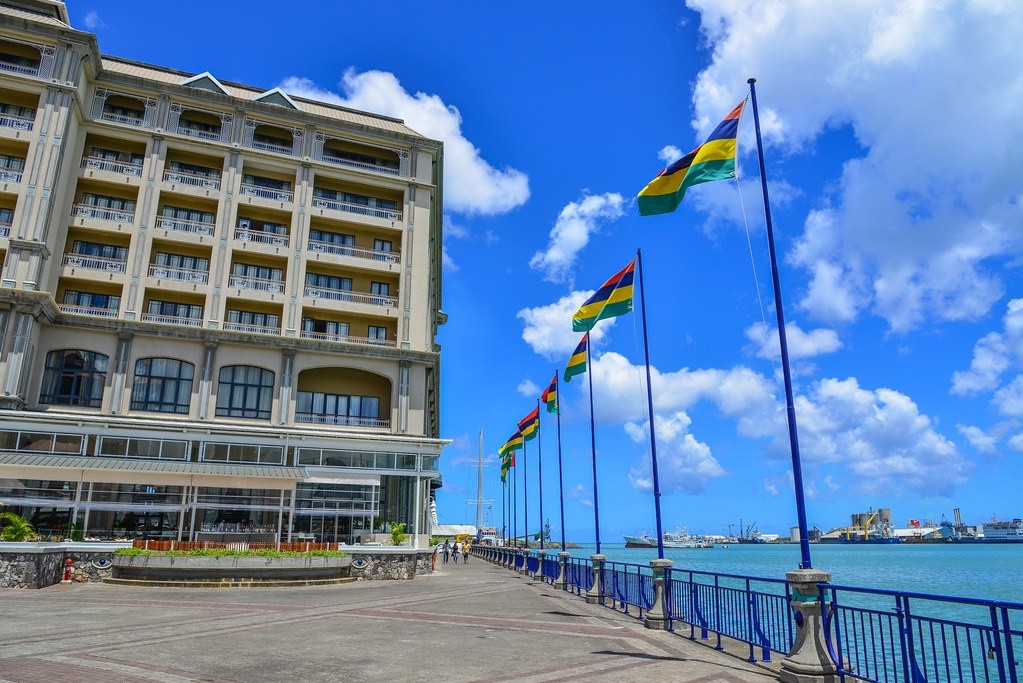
[437,543,448,553]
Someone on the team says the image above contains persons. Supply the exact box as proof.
[451,542,458,563]
[443,540,451,563]
[461,540,471,564]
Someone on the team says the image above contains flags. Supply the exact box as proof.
[636,96,748,217]
[572,257,637,332]
[497,430,524,458]
[517,407,540,441]
[542,377,558,414]
[564,333,587,382]
[500,452,515,482]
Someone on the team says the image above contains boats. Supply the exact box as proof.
[623,528,666,548]
[956,516,1023,544]
[714,524,740,544]
[737,518,767,544]
[646,525,715,548]
